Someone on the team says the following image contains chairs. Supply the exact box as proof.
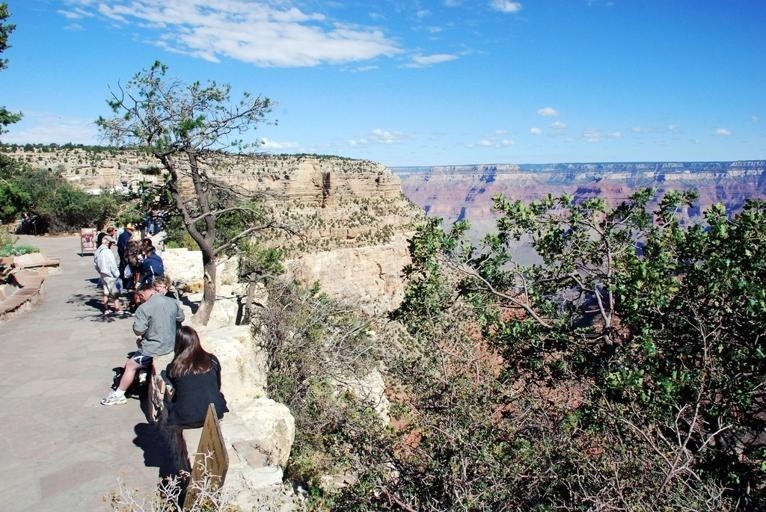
[80,227,97,257]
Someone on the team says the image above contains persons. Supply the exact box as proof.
[94,278,184,406]
[155,277,175,298]
[144,204,163,239]
[153,324,230,428]
[93,221,164,317]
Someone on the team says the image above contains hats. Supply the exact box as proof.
[122,223,136,231]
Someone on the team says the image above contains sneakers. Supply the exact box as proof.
[99,391,128,407]
